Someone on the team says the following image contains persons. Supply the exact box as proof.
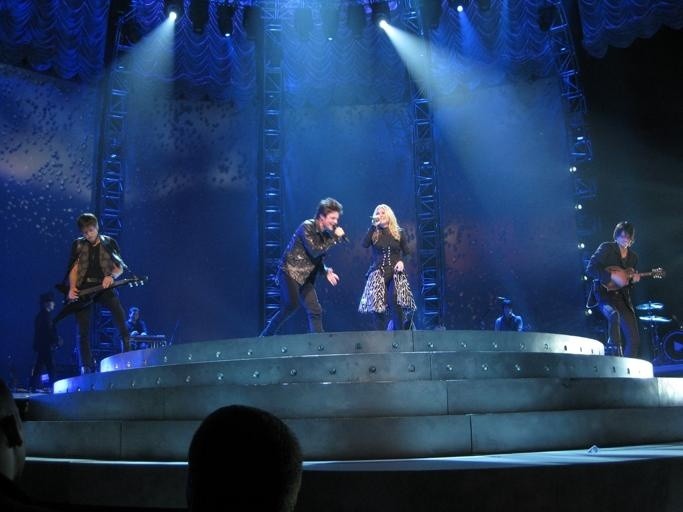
[125,306,148,349]
[255,195,345,337]
[25,292,63,390]
[356,203,417,331]
[186,402,305,510]
[584,220,642,358]
[492,296,524,332]
[65,212,130,376]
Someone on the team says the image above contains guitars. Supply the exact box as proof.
[601,266,665,291]
[53,275,148,324]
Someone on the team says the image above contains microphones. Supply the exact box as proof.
[333,224,349,243]
[369,215,380,220]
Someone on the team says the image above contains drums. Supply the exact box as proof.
[661,330,683,362]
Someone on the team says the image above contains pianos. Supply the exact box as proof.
[129,335,167,342]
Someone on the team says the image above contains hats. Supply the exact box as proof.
[38,292,55,304]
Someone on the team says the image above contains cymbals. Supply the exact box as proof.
[636,303,664,310]
[639,316,672,322]
